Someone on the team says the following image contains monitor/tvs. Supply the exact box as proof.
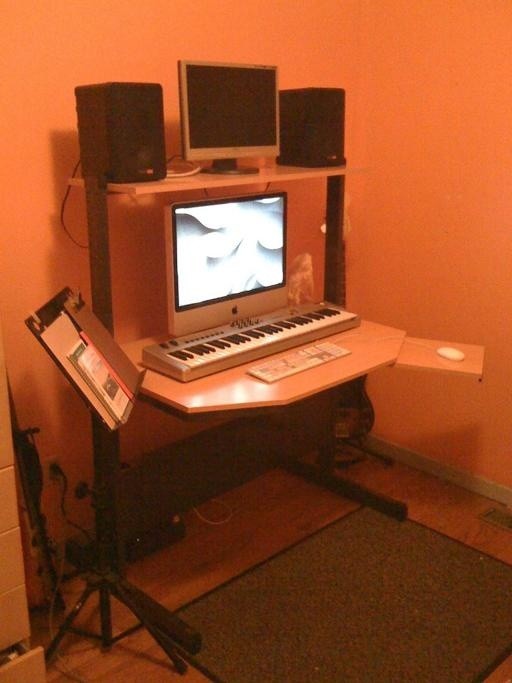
[162,189,288,338]
[177,58,279,175]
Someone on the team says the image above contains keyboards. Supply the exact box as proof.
[246,342,351,384]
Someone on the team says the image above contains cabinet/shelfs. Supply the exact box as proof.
[0,345,46,682]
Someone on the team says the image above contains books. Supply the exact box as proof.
[68,339,136,429]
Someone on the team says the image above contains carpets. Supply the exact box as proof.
[169,502,511,681]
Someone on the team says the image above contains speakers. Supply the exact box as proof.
[74,82,166,183]
[276,86,346,168]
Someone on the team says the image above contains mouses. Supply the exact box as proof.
[437,346,465,362]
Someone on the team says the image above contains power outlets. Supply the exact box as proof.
[48,461,60,480]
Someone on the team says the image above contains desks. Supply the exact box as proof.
[64,85,488,651]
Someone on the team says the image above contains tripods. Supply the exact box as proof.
[43,425,188,676]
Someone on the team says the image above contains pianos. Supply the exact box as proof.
[141,298,361,381]
